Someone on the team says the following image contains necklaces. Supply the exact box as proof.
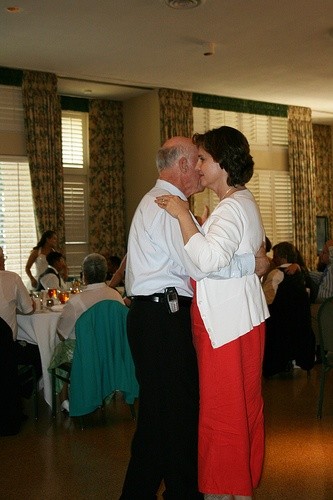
[220,184,239,201]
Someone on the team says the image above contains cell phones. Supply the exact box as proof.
[165,287,179,313]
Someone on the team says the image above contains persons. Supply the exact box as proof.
[261,236,333,376]
[37,252,67,292]
[0,246,37,437]
[47,253,126,413]
[119,137,270,500]
[154,126,273,500]
[26,231,58,290]
[106,256,122,280]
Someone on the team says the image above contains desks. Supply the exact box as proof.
[16,311,61,407]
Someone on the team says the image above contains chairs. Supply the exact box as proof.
[0,317,42,425]
[317,297,333,417]
[50,299,136,430]
[266,272,315,375]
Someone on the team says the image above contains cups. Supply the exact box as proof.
[28,277,81,313]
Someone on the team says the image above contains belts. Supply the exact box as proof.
[130,294,165,303]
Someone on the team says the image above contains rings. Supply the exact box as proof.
[162,201,165,206]
